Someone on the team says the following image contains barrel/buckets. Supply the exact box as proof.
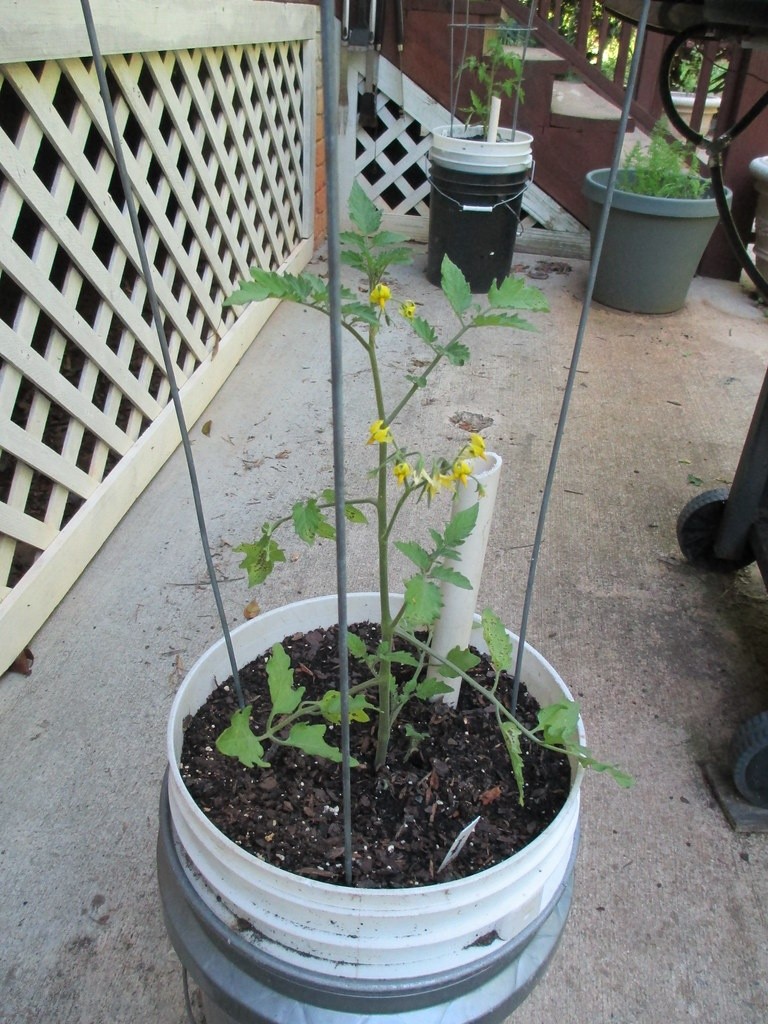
[426,160,529,294]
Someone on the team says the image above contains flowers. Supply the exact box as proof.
[193,176,638,812]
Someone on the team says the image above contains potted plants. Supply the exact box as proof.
[585,111,734,315]
